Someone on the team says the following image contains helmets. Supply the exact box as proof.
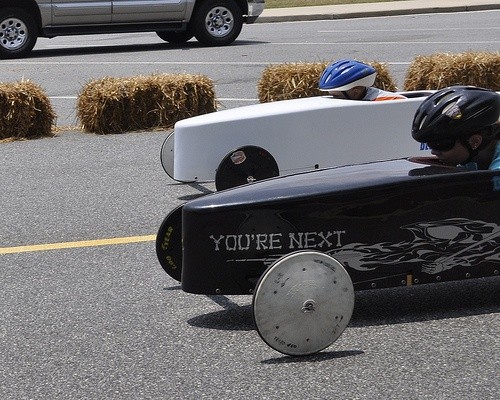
[318,59,377,91]
[411,85,500,144]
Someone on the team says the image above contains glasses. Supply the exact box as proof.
[427,139,457,152]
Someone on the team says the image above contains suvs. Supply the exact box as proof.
[0,0,268,59]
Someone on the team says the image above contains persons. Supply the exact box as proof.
[411,85,500,170]
[318,58,408,101]
